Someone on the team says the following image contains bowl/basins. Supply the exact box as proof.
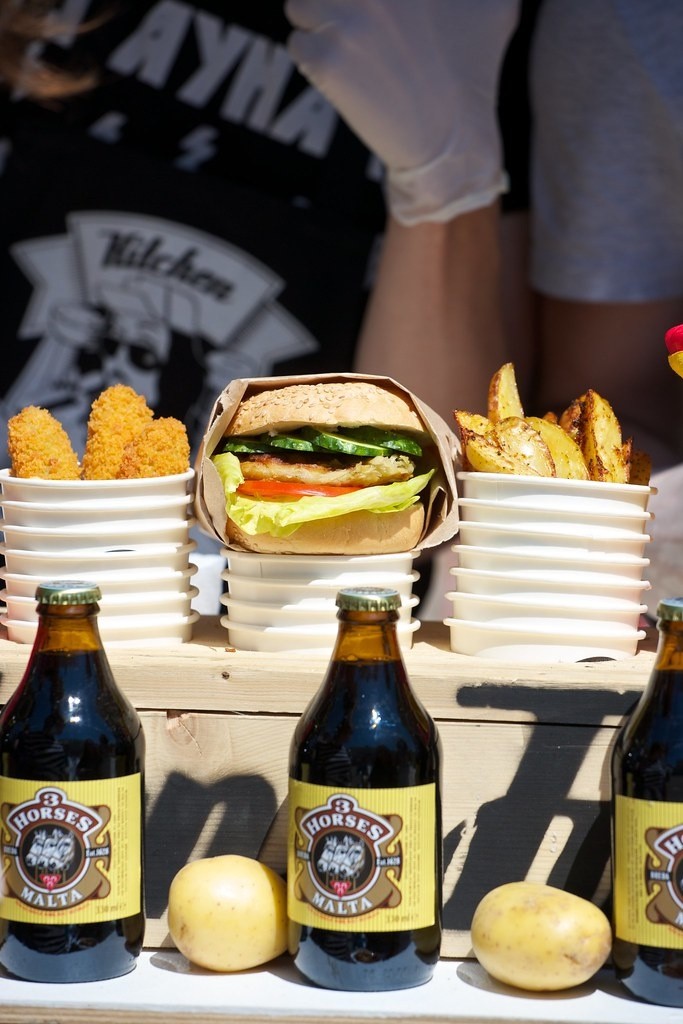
[1,464,204,661]
[221,521,424,661]
[442,475,655,669]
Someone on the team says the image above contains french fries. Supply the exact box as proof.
[455,360,652,487]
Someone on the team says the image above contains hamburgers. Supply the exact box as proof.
[212,381,440,557]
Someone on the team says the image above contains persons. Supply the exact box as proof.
[0,0,683,473]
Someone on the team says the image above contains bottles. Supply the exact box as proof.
[606,596,683,1009]
[1,578,150,984]
[285,586,444,992]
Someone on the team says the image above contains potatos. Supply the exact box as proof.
[471,881,612,990]
[166,855,288,971]
